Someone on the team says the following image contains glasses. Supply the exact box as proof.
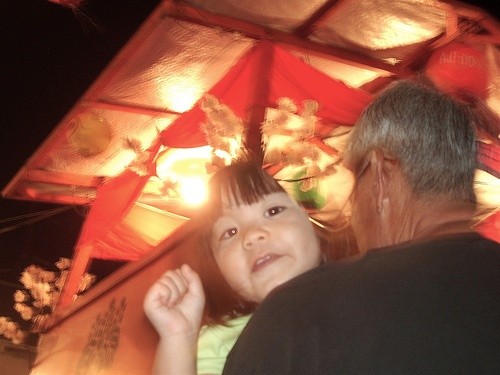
[339,156,396,221]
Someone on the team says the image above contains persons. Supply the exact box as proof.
[141,160,325,375]
[222,77,500,375]
